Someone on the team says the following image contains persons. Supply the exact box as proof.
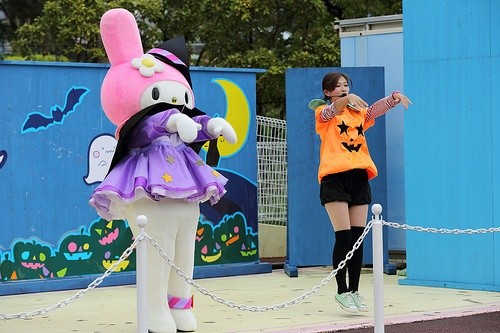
[314,71,412,313]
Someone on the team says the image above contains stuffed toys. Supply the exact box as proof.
[87,8,237,333]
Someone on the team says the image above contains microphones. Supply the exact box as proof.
[326,92,347,100]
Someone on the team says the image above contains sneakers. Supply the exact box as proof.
[335,291,358,312]
[352,292,368,312]
